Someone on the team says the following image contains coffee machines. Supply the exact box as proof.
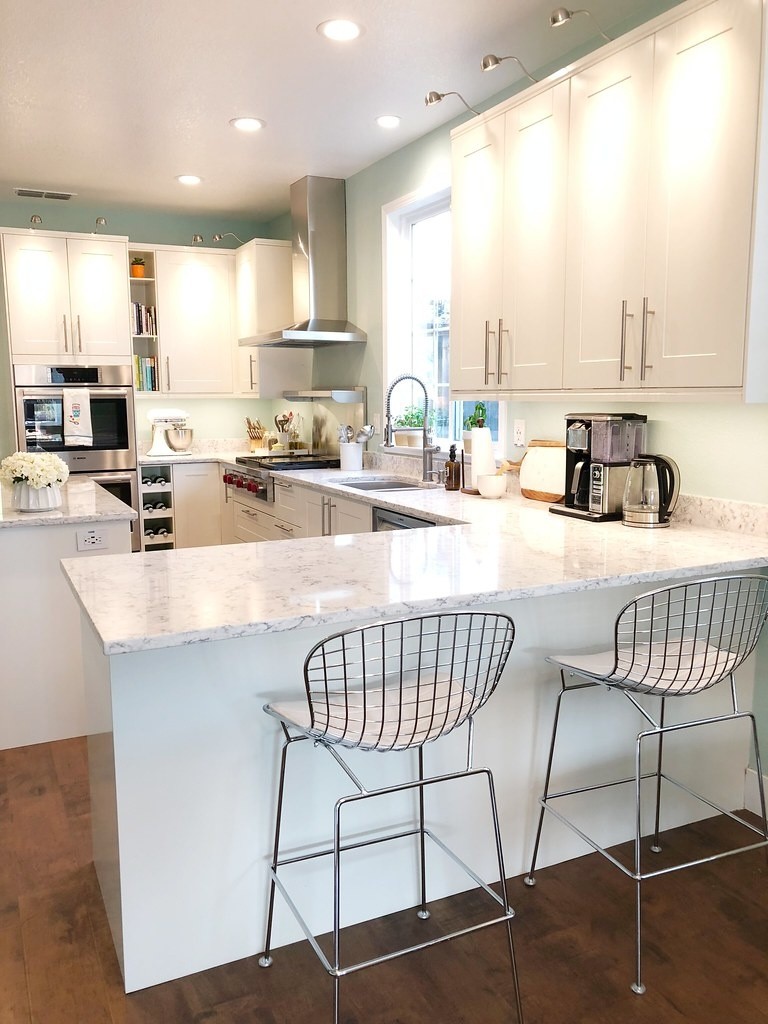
[564,412,648,513]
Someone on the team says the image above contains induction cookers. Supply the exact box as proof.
[221,454,340,503]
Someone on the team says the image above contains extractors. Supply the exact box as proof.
[237,176,367,348]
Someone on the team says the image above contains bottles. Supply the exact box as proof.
[143,475,166,486]
[262,431,278,451]
[444,444,460,491]
[145,527,168,538]
[144,502,166,512]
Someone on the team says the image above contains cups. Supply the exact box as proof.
[288,441,299,454]
[339,442,363,470]
[278,433,289,450]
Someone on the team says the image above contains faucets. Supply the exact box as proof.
[382,375,441,483]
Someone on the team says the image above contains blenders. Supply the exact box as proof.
[147,408,191,457]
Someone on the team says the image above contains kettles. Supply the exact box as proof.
[622,453,680,528]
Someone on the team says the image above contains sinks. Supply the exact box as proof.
[328,474,446,496]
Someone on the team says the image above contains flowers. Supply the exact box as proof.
[0,449,70,490]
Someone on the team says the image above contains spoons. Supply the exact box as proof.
[355,424,375,443]
[345,425,354,443]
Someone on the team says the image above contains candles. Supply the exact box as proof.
[462,400,486,453]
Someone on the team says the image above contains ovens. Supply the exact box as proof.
[13,364,142,553]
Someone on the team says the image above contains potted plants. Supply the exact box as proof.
[391,407,436,447]
[130,257,145,278]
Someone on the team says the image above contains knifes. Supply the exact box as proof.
[245,417,263,440]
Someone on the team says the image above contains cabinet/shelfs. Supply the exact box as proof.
[139,464,374,552]
[0,227,294,399]
[448,0,768,399]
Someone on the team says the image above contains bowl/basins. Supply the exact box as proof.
[165,429,193,452]
[476,474,507,499]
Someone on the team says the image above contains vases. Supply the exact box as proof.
[11,478,62,513]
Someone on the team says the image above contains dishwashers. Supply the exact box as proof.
[372,506,435,532]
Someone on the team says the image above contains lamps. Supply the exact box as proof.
[550,8,611,42]
[481,54,537,83]
[192,235,203,246]
[213,233,245,244]
[95,217,107,234]
[31,215,42,229]
[426,92,481,115]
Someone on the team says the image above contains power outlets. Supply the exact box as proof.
[76,529,108,551]
[514,419,525,445]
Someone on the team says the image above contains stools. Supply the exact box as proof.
[522,575,768,993]
[258,610,524,1024]
